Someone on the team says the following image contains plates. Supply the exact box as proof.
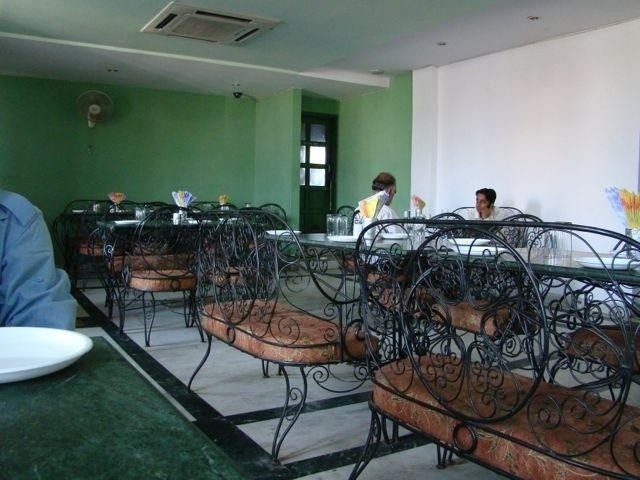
[447,237,491,246]
[453,246,503,256]
[0,325,95,387]
[573,255,640,271]
[266,229,302,236]
[72,209,89,213]
[610,248,627,258]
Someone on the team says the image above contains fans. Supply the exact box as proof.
[77,90,115,129]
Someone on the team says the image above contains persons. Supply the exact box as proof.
[353,172,415,283]
[1,187,78,332]
[453,186,518,250]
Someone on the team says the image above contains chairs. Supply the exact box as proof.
[50,198,289,348]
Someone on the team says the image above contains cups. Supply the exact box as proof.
[92,203,253,225]
[324,209,449,242]
[624,227,639,260]
